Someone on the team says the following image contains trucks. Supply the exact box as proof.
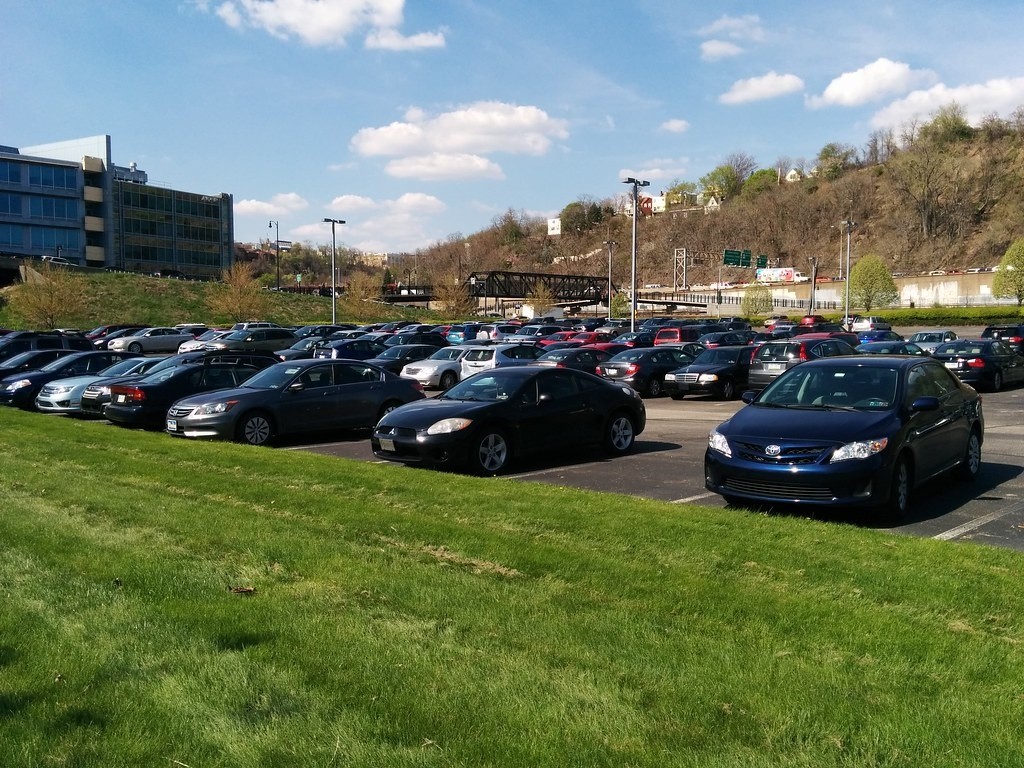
[756,268,811,283]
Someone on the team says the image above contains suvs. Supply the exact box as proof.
[230,322,281,329]
[0,332,91,363]
[206,328,299,355]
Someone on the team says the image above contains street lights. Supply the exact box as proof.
[322,218,346,324]
[841,220,858,325]
[268,220,279,292]
[621,178,651,333]
[602,241,619,321]
[808,257,818,315]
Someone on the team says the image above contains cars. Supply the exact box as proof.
[372,366,647,475]
[153,269,190,280]
[705,354,984,521]
[9,255,79,266]
[107,327,196,353]
[1,314,1024,531]
[164,359,426,446]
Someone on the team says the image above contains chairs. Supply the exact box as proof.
[630,357,637,360]
[946,348,955,354]
[856,383,889,408]
[881,349,889,353]
[622,357,629,361]
[219,373,234,385]
[204,375,213,385]
[320,373,331,385]
[972,349,980,353]
[811,389,850,406]
[298,374,311,383]
